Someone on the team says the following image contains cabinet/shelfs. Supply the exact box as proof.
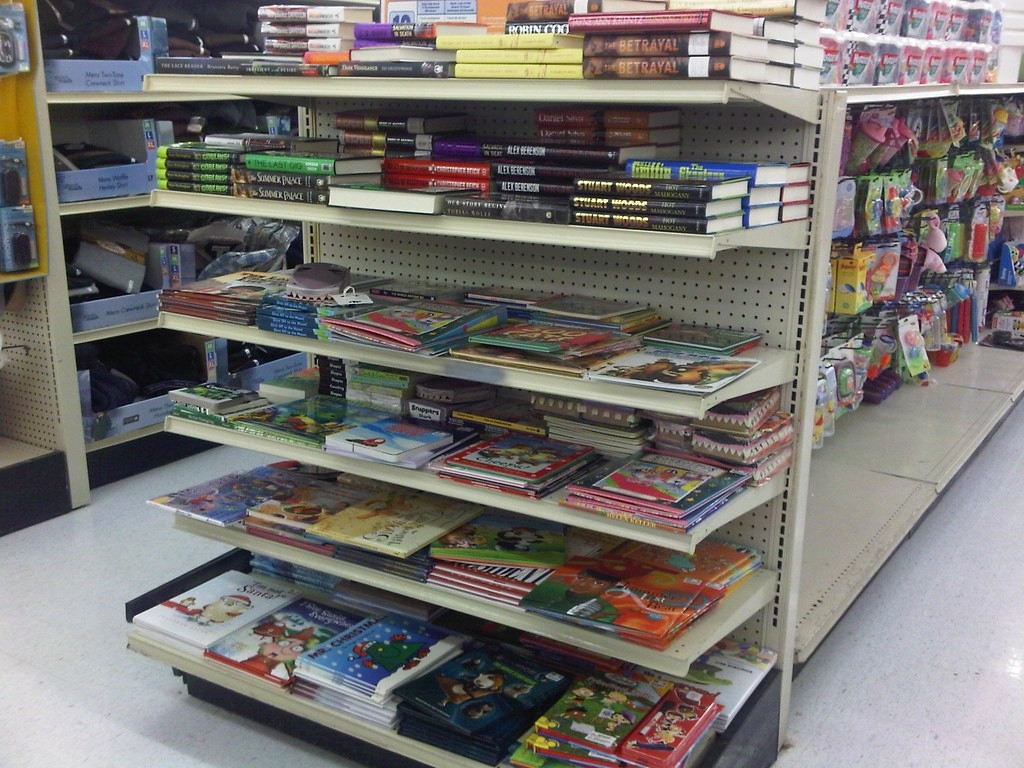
[1,1,314,537]
[124,74,846,767]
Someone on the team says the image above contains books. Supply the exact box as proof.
[126,356,793,767]
[153,0,830,398]
